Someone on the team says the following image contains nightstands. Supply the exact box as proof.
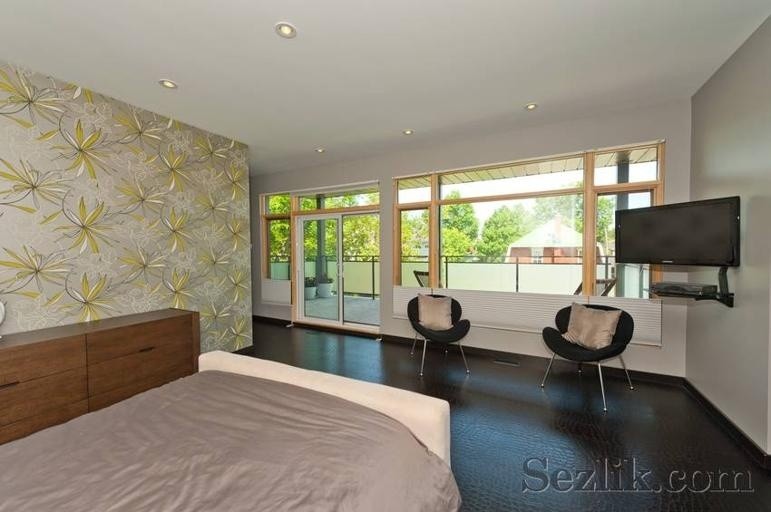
[88,308,201,414]
[0,322,88,446]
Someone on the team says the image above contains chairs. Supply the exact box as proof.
[414,271,444,288]
[540,304,636,413]
[408,294,472,377]
[572,280,618,296]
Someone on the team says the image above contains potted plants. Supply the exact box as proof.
[316,278,334,298]
[304,277,319,300]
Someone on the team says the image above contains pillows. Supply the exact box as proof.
[561,301,624,351]
[418,293,454,330]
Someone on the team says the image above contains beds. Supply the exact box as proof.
[0,349,463,510]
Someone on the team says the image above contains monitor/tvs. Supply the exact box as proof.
[615,195,741,265]
